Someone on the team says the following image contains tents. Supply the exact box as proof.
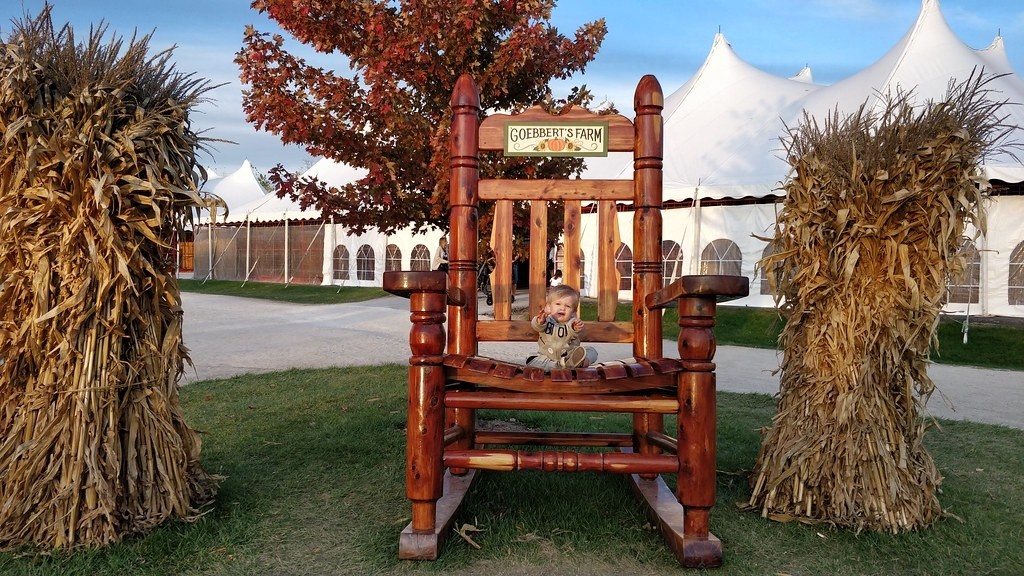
[175,0,1024,319]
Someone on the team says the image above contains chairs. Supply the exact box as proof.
[382,73,749,566]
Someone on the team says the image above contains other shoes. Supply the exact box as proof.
[556,357,565,370]
[565,346,586,368]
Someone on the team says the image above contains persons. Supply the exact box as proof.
[526,285,598,372]
[511,241,519,303]
[432,237,449,274]
[549,270,563,290]
